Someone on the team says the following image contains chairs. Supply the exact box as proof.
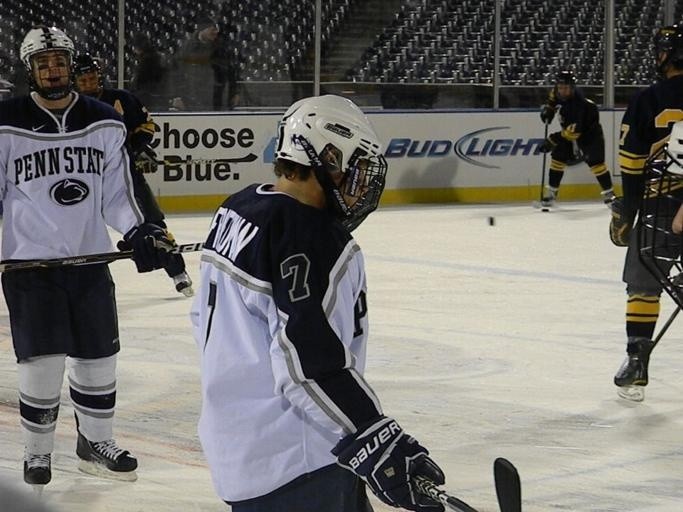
[340,1,665,83]
[3,1,351,84]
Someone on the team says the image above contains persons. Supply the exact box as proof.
[68,49,194,294]
[189,91,449,512]
[537,73,617,213]
[0,25,176,486]
[176,17,240,111]
[606,18,683,389]
[126,30,173,113]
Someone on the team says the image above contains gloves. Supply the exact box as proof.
[541,107,554,124]
[539,134,559,153]
[331,416,444,512]
[610,197,637,246]
[117,224,174,272]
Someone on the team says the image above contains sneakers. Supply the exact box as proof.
[533,197,556,208]
[615,357,648,386]
[173,272,192,290]
[76,432,137,471]
[23,454,51,484]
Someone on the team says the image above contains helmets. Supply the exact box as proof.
[654,26,683,52]
[555,70,576,85]
[20,26,100,100]
[274,94,388,231]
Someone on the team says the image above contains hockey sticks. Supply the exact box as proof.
[410,457,522,511]
[132,153,258,166]
[532,117,549,209]
